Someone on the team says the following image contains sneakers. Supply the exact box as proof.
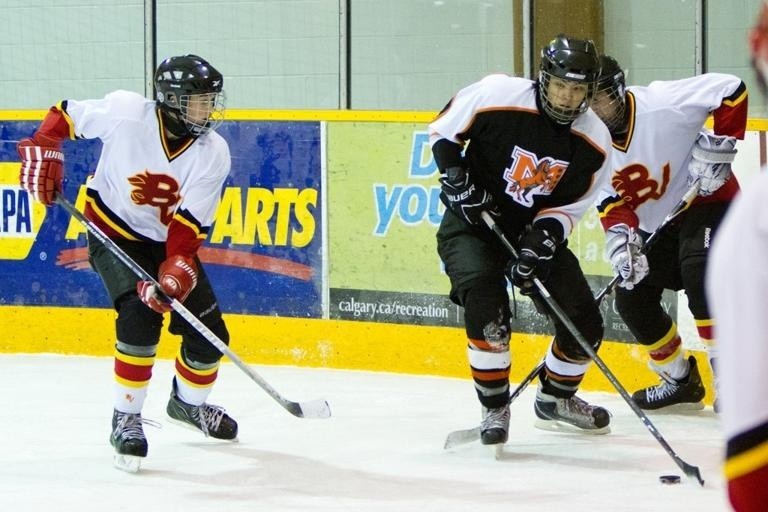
[533,366,610,430]
[631,354,706,410]
[166,373,238,441]
[709,356,722,415]
[109,407,148,459]
[480,400,512,446]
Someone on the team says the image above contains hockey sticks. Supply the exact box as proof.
[52,190,331,418]
[444,181,701,449]
[482,210,705,486]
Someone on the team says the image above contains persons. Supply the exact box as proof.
[17,52,239,458]
[425,32,614,446]
[588,52,748,412]
[705,1,768,511]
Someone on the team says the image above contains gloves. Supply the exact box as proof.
[504,228,558,286]
[17,132,65,208]
[438,167,502,227]
[686,131,738,198]
[602,222,651,291]
[136,254,200,314]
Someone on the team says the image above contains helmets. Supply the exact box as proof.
[538,34,601,120]
[582,54,627,133]
[153,54,224,139]
